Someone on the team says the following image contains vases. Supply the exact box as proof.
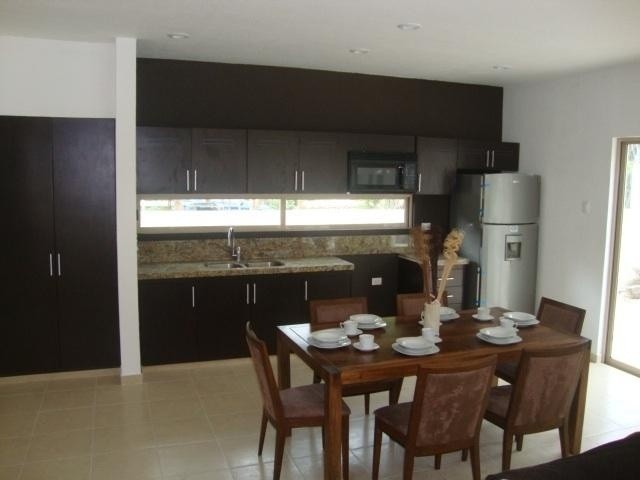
[419,300,441,335]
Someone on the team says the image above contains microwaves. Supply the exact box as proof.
[349,154,420,195]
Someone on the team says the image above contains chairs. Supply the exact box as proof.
[308,298,405,415]
[243,321,352,479]
[489,297,586,440]
[476,339,590,473]
[397,288,449,321]
[372,348,499,480]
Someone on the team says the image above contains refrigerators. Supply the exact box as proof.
[449,171,541,319]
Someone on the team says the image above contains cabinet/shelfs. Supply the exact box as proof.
[398,255,470,310]
[135,123,247,200]
[0,115,122,377]
[347,134,416,195]
[461,142,518,174]
[248,127,347,199]
[138,276,202,369]
[415,138,461,195]
[203,273,282,362]
[281,270,354,353]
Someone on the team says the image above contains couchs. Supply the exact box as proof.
[485,430,640,480]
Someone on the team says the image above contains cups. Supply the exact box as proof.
[359,334,376,349]
[421,222,432,231]
[340,321,358,334]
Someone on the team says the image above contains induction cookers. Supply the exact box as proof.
[337,254,413,266]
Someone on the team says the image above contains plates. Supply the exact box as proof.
[308,329,352,349]
[350,313,386,330]
[391,303,539,357]
[347,330,363,338]
[352,343,382,351]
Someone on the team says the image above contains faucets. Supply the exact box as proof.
[226,226,235,256]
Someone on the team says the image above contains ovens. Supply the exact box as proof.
[355,268,421,317]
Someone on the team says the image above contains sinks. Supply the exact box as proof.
[204,260,243,270]
[244,259,285,268]
